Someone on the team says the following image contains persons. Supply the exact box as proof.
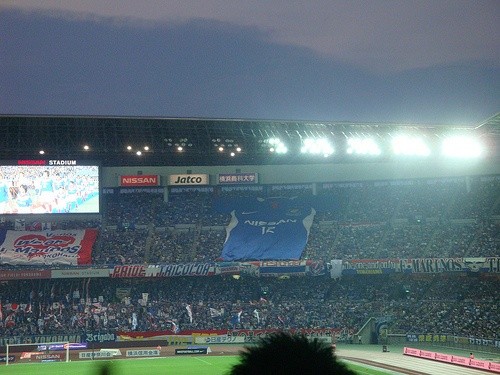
[332,341,337,351]
[1,165,100,214]
[157,344,162,351]
[469,352,474,359]
[228,331,356,374]
[0,185,498,340]
